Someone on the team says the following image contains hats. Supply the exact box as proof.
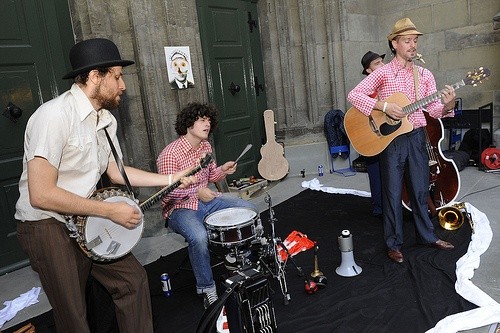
[361,51,386,75]
[62,38,134,80]
[387,18,423,42]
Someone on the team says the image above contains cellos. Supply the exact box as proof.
[400,107,462,213]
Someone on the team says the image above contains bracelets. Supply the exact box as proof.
[383,101,387,112]
[168,174,172,186]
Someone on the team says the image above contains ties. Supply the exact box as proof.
[182,83,185,88]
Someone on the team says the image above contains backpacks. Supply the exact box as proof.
[442,150,472,172]
[459,129,490,160]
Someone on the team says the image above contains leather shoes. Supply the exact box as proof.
[434,236,454,249]
[387,248,404,263]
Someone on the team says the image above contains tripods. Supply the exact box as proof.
[255,184,308,305]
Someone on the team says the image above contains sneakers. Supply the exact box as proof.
[204,289,218,309]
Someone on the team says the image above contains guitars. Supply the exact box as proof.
[343,66,493,158]
[71,147,217,264]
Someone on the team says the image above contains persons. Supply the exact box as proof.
[361,51,386,216]
[347,17,455,263]
[14,37,198,333]
[156,102,260,311]
[169,49,195,90]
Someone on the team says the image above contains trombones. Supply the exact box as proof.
[437,200,475,235]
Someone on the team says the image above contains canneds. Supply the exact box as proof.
[318,165,323,176]
[160,273,172,295]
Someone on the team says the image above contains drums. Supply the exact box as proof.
[203,205,263,247]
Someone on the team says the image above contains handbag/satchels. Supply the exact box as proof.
[478,145,500,171]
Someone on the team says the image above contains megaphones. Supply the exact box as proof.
[336,230,363,277]
